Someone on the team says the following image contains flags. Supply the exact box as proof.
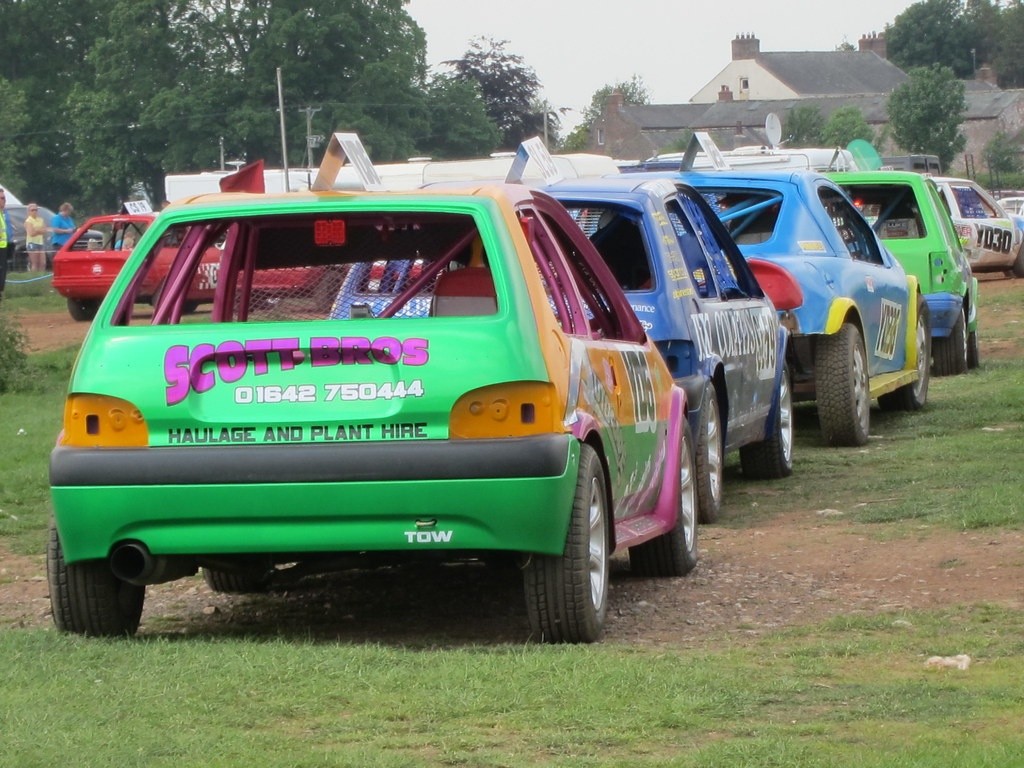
[218,160,266,194]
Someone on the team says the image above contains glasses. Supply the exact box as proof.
[0,197,5,200]
[30,209,37,212]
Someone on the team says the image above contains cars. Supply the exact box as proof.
[5,203,106,275]
[615,128,935,447]
[996,196,1024,230]
[407,135,798,525]
[51,201,229,324]
[727,137,979,376]
[920,171,1024,279]
[48,134,699,640]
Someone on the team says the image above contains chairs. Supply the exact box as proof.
[879,202,924,238]
[430,266,498,316]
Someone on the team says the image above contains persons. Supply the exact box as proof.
[115,232,138,251]
[23,204,48,272]
[51,203,77,251]
[0,187,15,302]
